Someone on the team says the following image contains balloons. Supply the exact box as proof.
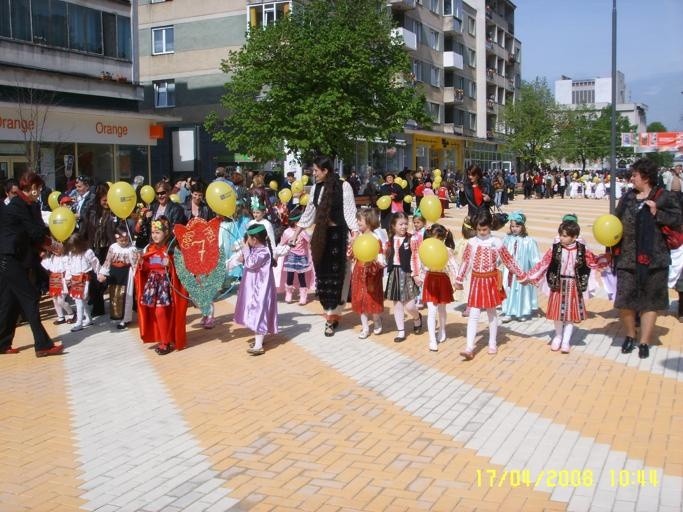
[593,213,623,255]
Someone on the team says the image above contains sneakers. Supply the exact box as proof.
[3,310,214,357]
[324,302,570,358]
[247,348,264,355]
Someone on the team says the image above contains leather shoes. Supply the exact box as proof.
[621,335,636,353]
[638,343,649,359]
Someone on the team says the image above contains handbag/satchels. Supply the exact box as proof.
[659,224,683,250]
[490,213,508,231]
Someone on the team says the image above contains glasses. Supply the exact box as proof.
[154,190,168,195]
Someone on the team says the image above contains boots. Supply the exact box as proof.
[284,283,295,303]
[298,287,308,305]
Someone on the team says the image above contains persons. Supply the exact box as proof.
[132,214,188,355]
[273,210,313,306]
[200,304,216,329]
[495,209,542,320]
[346,208,386,338]
[280,157,358,338]
[39,243,75,325]
[62,233,104,332]
[64,182,125,319]
[541,215,600,302]
[232,224,279,355]
[1,155,515,270]
[523,160,682,200]
[380,211,424,343]
[605,157,678,358]
[416,224,457,353]
[523,219,609,354]
[0,173,65,357]
[98,224,143,330]
[453,211,529,359]
